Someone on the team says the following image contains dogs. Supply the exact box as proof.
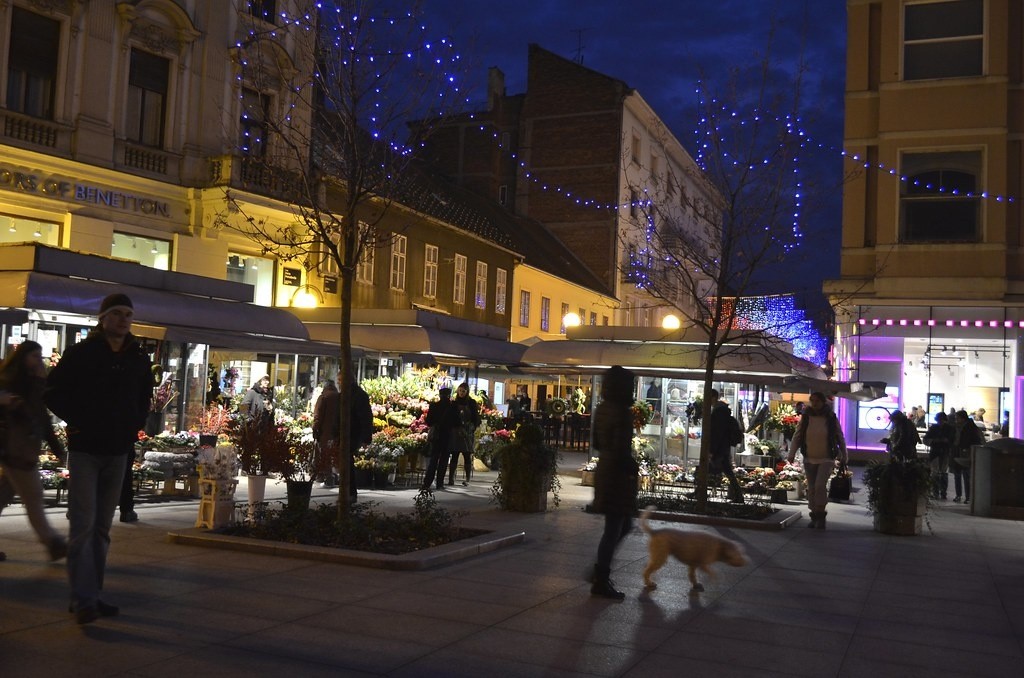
[639,505,747,589]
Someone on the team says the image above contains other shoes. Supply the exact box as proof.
[953,496,962,501]
[684,493,696,499]
[449,481,454,485]
[263,471,276,479]
[69,599,119,624]
[930,493,939,500]
[120,512,137,522]
[241,471,251,476]
[0,552,7,562]
[941,493,947,499]
[325,480,335,487]
[729,498,745,503]
[436,487,446,490]
[49,537,68,561]
[463,482,469,486]
[964,498,971,504]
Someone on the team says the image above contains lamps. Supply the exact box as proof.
[226,255,258,269]
[921,345,1010,378]
[8,218,41,238]
[289,283,323,308]
[112,235,157,254]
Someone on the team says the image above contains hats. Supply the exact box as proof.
[253,372,269,383]
[439,388,452,395]
[99,293,134,319]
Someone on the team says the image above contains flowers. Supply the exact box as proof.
[548,388,853,491]
[42,351,512,480]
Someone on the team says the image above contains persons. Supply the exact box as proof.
[447,383,482,486]
[794,400,807,417]
[788,392,848,532]
[505,391,590,440]
[244,374,275,424]
[417,388,456,491]
[645,381,674,416]
[0,339,70,562]
[583,369,640,600]
[686,389,744,503]
[119,443,138,521]
[43,293,155,625]
[880,410,922,463]
[309,369,373,502]
[909,405,1009,504]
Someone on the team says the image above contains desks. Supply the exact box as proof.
[734,453,776,469]
[507,412,591,451]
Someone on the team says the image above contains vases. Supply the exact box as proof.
[327,454,430,491]
[247,474,265,524]
[286,481,313,518]
[200,435,217,445]
[194,465,239,531]
[582,471,595,486]
[770,490,788,503]
[785,481,803,500]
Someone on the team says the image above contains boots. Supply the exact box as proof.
[808,512,827,528]
[591,563,625,599]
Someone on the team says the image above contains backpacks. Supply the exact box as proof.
[729,416,744,447]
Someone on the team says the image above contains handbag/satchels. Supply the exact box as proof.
[828,464,852,501]
[308,439,320,481]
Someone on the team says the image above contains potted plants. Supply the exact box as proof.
[861,453,947,537]
[491,422,561,512]
